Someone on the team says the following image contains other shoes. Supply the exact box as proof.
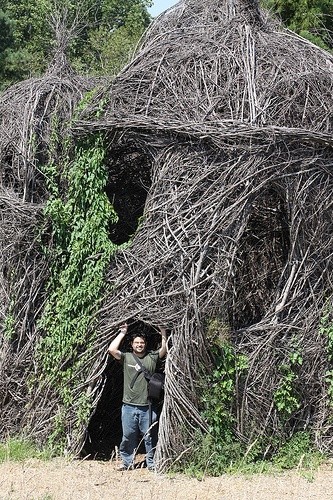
[148,466,155,473]
[117,464,132,471]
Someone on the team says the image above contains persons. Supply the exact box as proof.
[108,323,167,471]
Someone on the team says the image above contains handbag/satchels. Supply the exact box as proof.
[149,372,164,402]
